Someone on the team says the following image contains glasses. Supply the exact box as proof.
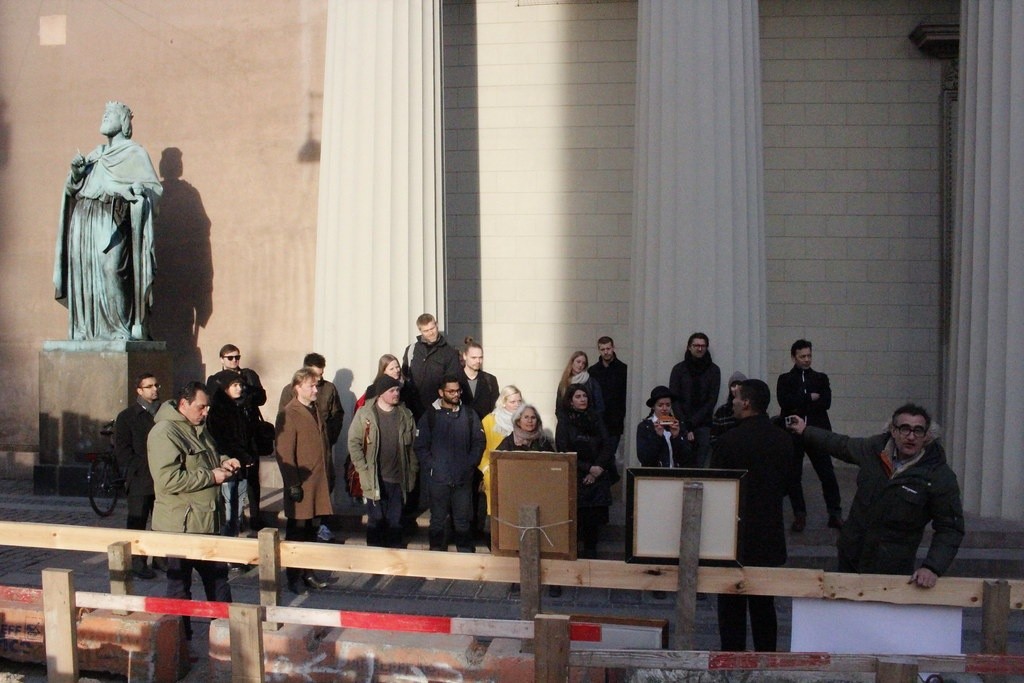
[138,383,162,390]
[690,344,706,349]
[222,355,241,361]
[731,380,742,387]
[444,388,462,396]
[891,423,926,439]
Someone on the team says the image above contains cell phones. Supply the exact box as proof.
[660,416,675,425]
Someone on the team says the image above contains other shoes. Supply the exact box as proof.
[550,585,562,598]
[653,590,666,599]
[511,583,520,591]
[696,592,708,600]
[186,641,199,663]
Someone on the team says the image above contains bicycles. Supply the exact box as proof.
[83,420,132,517]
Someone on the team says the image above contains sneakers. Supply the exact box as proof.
[827,515,845,528]
[318,525,335,540]
[151,558,168,571]
[791,517,806,531]
[132,566,157,579]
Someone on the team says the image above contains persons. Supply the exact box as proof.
[52,100,163,341]
[785,403,965,588]
[115,344,345,663]
[347,313,628,599]
[633,333,842,651]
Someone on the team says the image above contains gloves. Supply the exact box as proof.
[290,486,303,504]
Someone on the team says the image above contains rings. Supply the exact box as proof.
[591,482,593,483]
[588,481,590,482]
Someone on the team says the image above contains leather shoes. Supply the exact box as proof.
[288,582,317,596]
[306,576,329,589]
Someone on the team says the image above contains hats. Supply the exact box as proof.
[375,373,399,396]
[213,370,240,391]
[727,371,747,390]
[646,386,676,407]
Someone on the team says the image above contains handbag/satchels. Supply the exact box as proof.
[255,419,275,456]
[344,454,363,497]
